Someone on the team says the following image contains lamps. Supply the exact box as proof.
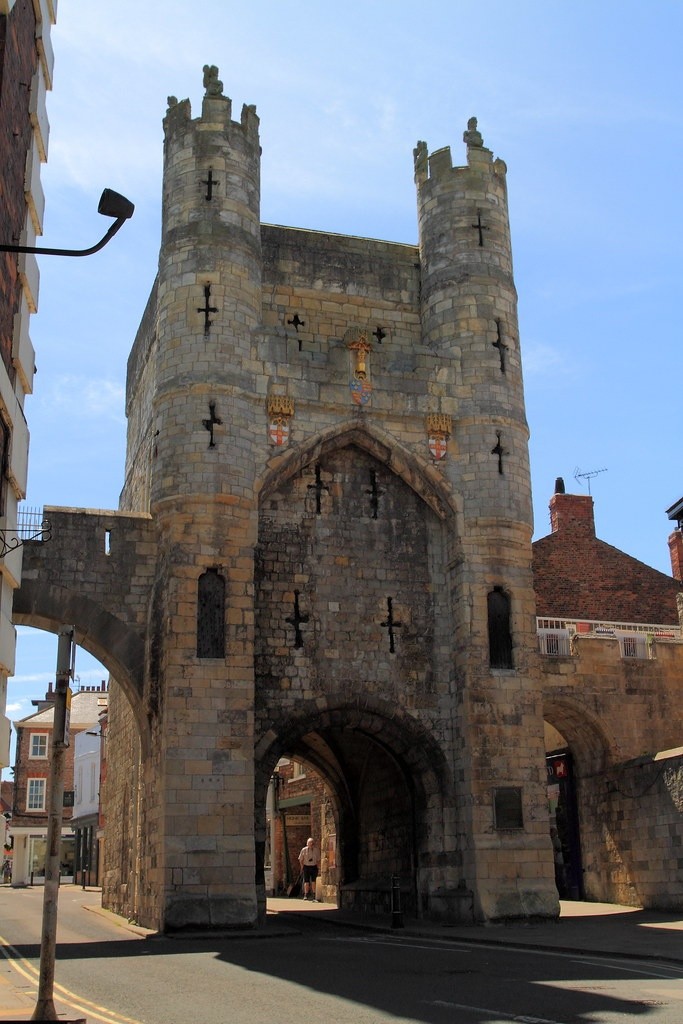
[86,731,107,738]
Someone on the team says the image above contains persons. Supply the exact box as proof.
[299,838,321,901]
[0,859,12,884]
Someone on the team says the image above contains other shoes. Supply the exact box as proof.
[304,893,309,900]
[313,895,316,900]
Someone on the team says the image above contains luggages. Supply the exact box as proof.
[286,873,303,898]
